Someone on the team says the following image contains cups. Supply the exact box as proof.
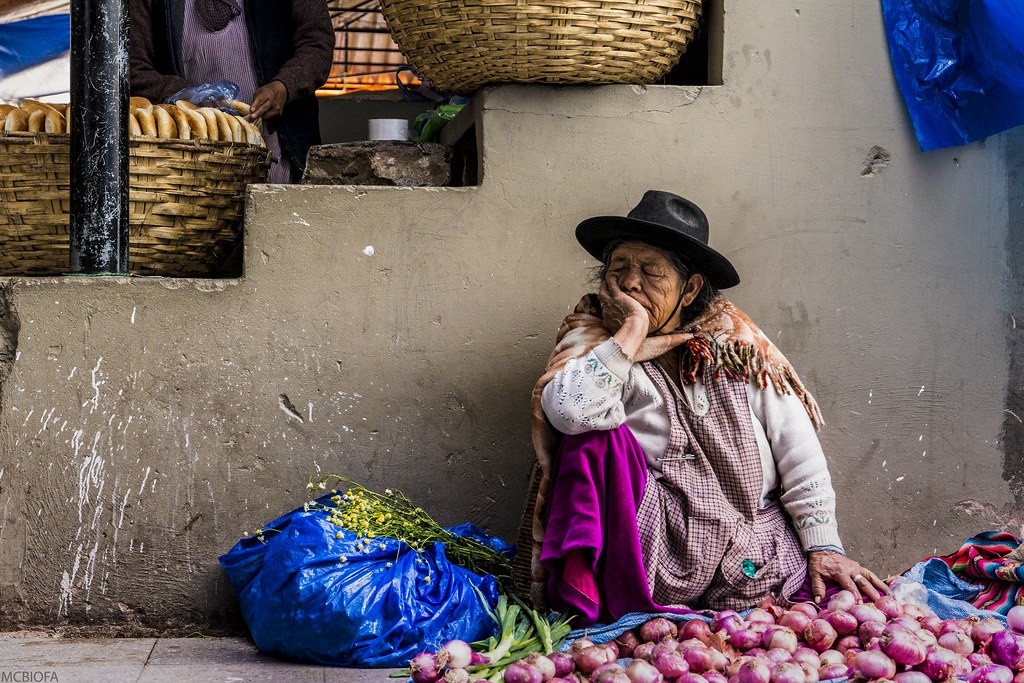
[369,119,408,140]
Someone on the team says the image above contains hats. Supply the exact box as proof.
[575,189,741,290]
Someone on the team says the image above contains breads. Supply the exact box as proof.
[0,96,266,147]
[315,71,421,98]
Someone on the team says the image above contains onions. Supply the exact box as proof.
[407,587,1024,683]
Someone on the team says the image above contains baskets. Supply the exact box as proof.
[378,0,704,93]
[0,129,274,275]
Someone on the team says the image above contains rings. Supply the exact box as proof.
[601,303,604,308]
[853,575,863,581]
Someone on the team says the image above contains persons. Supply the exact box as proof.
[127,0,336,183]
[533,189,892,621]
